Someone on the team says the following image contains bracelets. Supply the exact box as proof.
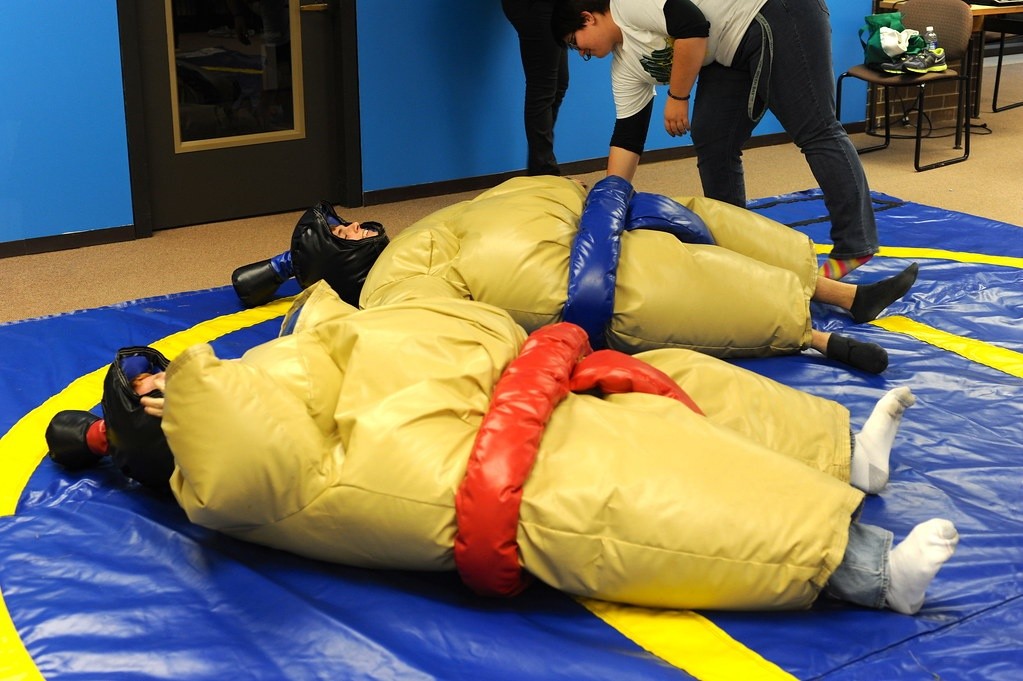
[667,88,690,100]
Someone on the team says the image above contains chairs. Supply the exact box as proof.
[980,12,1023,111]
[837,0,973,170]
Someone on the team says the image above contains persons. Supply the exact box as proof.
[501,0,589,191]
[551,0,881,281]
[291,173,921,376]
[100,298,960,616]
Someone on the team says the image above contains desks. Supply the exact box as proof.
[868,0,1023,149]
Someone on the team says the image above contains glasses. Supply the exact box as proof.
[567,31,576,50]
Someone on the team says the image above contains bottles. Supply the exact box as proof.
[925,25,938,51]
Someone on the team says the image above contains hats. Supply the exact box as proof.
[45,346,175,483]
[231,202,390,305]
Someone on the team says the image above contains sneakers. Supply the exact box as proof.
[881,54,915,72]
[906,47,948,72]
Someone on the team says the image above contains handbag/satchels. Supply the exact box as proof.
[864,26,926,70]
[858,11,906,49]
